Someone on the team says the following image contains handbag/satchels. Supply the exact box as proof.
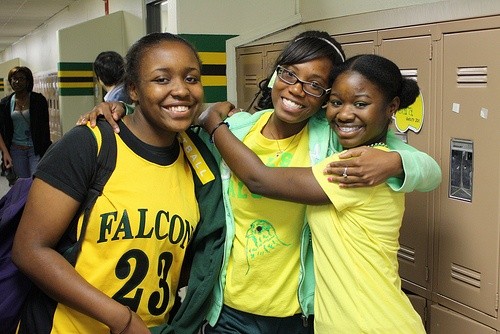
[0,115,122,334]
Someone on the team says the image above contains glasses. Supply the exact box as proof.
[277,65,332,99]
[10,76,25,82]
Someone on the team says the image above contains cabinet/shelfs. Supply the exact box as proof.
[32,68,63,143]
[226,1,500,334]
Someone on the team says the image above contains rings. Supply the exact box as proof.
[343,167,348,178]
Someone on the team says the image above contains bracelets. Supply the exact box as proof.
[110,306,132,334]
[210,122,230,143]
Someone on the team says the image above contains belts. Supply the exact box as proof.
[12,144,31,150]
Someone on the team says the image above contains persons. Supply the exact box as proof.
[8,32,205,334]
[94,51,127,103]
[200,54,429,334]
[0,66,53,188]
[76,31,444,334]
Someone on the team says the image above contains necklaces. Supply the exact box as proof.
[268,117,299,153]
[367,142,389,148]
[19,102,27,108]
